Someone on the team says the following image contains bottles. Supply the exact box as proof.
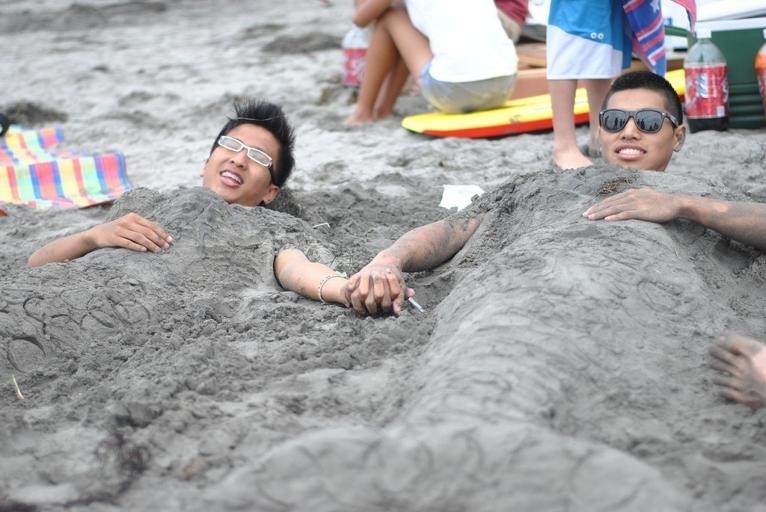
[754,30,765,114]
[684,26,731,134]
[342,22,370,89]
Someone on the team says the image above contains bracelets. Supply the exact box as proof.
[318,274,348,304]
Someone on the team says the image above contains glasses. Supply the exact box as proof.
[216,135,277,186]
[599,108,680,134]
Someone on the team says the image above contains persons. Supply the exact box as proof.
[495,1,530,45]
[27,100,415,303]
[340,71,766,315]
[708,333,766,408]
[344,1,519,125]
[545,0,634,170]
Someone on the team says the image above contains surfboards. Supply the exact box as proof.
[402,68,685,137]
